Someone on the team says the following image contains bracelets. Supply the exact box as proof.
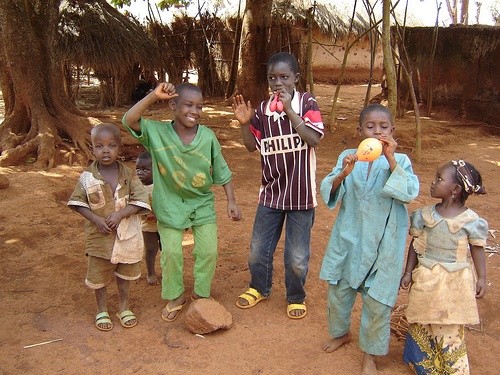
[291,115,299,122]
[405,271,411,275]
[294,121,304,129]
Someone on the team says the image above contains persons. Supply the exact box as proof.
[233,52,325,320]
[121,81,247,321]
[319,103,422,375]
[399,160,489,375]
[135,152,165,286]
[67,123,153,331]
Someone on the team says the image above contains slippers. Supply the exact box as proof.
[162,298,186,322]
[95,312,113,331]
[236,287,267,309]
[287,301,306,319]
[192,295,214,302]
[115,310,136,327]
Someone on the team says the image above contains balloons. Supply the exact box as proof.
[355,137,385,162]
[268,93,284,113]
